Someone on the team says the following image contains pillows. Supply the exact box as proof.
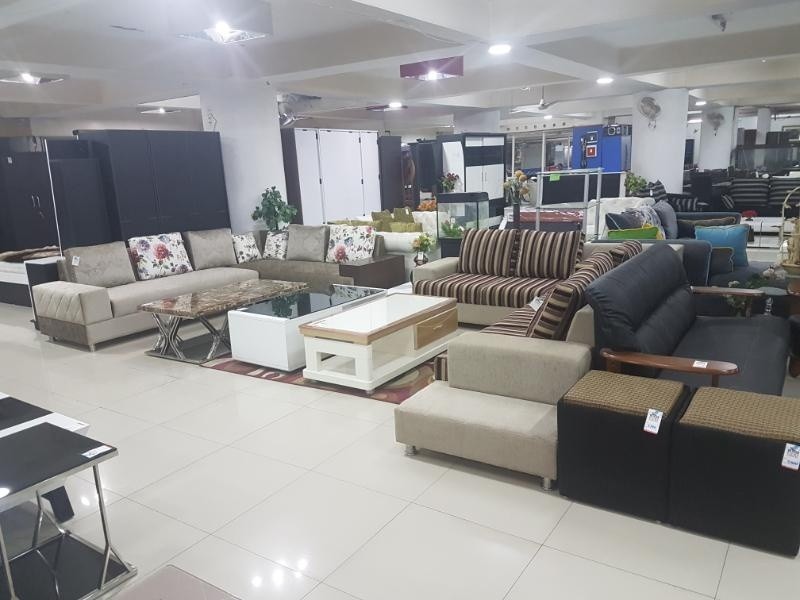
[351,208,423,232]
[604,180,679,240]
[516,229,587,279]
[720,179,769,210]
[457,229,520,277]
[608,226,663,240]
[695,224,749,267]
[770,177,800,207]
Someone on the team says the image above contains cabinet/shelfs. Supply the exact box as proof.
[408,128,508,229]
[0,130,231,254]
[281,128,404,225]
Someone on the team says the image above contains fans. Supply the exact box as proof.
[706,112,725,136]
[639,97,661,128]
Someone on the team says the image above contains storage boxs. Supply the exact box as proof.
[436,192,489,243]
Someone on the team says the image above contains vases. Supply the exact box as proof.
[513,204,520,229]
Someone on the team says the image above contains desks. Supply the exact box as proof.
[227,284,387,373]
[138,279,309,365]
[0,392,137,600]
[299,293,458,394]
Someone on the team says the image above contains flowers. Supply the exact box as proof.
[503,170,529,203]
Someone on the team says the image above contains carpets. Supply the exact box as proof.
[201,352,436,404]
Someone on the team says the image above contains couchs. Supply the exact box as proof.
[32,229,800,560]
